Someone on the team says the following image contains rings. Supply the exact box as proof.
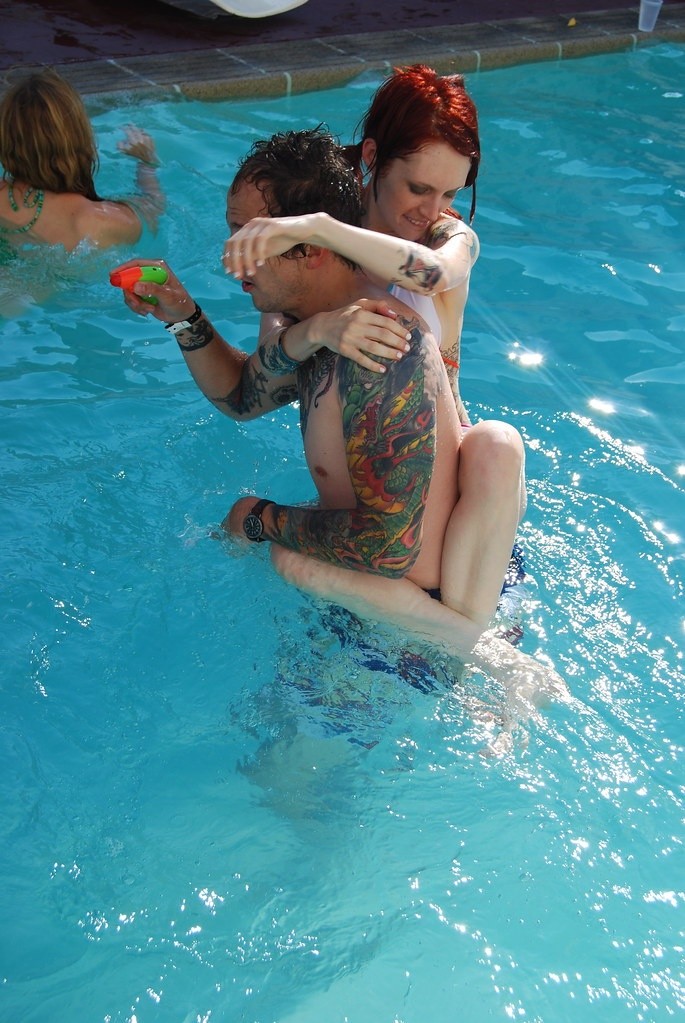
[221,252,229,260]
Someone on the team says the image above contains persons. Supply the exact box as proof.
[221,64,527,615]
[110,131,461,587]
[0,73,142,251]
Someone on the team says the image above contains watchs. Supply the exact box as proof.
[243,499,277,542]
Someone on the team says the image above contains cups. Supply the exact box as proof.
[636,0,664,32]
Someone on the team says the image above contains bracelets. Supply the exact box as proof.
[278,330,305,367]
[165,301,201,334]
